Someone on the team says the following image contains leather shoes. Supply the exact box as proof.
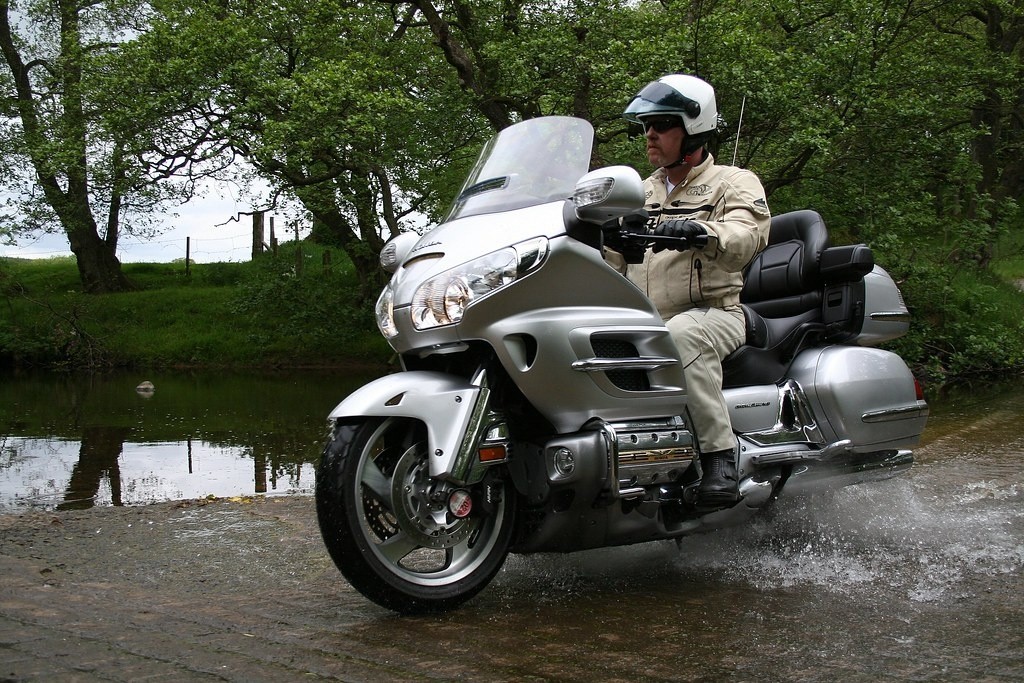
[694,448,739,504]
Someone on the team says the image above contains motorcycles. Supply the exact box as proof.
[314,116,930,620]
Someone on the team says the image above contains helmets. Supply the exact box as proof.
[635,74,717,155]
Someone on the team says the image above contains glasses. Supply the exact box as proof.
[642,117,687,133]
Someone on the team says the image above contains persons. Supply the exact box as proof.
[604,74,771,508]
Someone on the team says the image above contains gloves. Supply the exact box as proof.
[651,220,708,253]
